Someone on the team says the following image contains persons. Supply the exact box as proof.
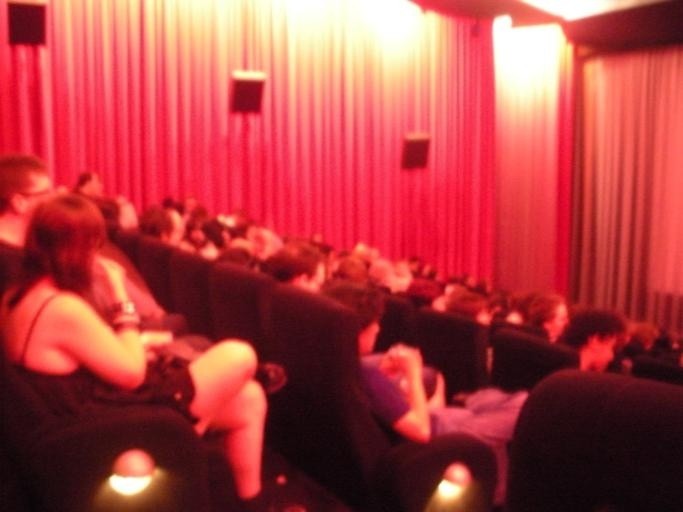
[2,153,683,512]
